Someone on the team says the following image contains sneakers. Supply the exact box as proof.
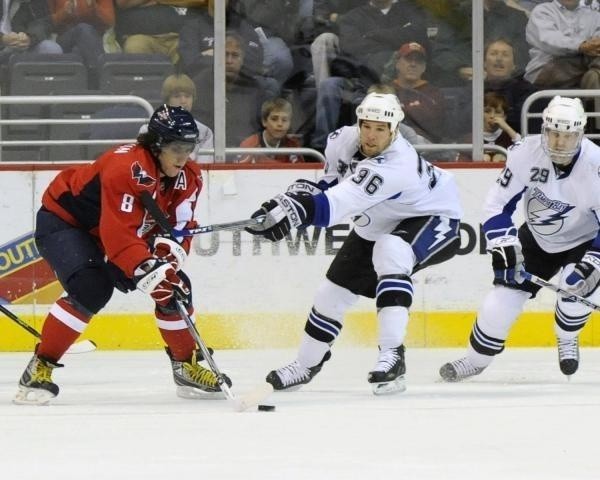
[266,351,330,388]
[555,334,580,374]
[165,345,232,391]
[440,358,483,379]
[18,343,63,396]
[368,345,406,381]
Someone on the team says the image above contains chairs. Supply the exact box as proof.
[0,1,600,167]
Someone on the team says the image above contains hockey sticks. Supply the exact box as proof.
[0,304,98,354]
[140,189,266,238]
[174,297,276,413]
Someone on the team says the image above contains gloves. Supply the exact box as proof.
[246,179,319,242]
[487,228,524,284]
[561,251,600,299]
[134,238,189,311]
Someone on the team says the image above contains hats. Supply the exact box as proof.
[401,42,425,56]
[160,75,197,104]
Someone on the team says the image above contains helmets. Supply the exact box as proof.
[540,96,587,164]
[355,91,404,130]
[147,104,201,158]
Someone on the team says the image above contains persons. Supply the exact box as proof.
[234,1,600,164]
[14,105,234,394]
[139,30,303,165]
[439,93,599,382]
[1,1,264,89]
[245,89,460,394]
